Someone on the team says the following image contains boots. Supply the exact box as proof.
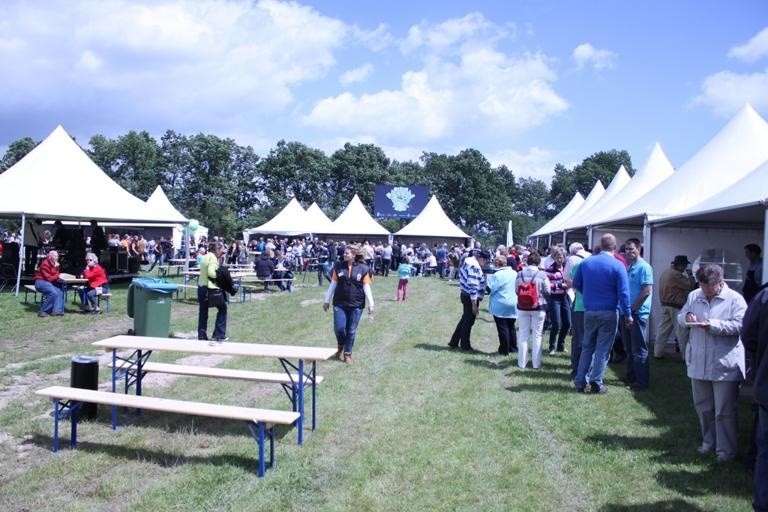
[342,351,353,364]
[336,347,343,359]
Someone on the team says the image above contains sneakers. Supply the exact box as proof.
[211,337,228,342]
[590,385,609,394]
[549,348,556,356]
[38,311,48,317]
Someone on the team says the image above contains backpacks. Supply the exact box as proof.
[518,272,539,309]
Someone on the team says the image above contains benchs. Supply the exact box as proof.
[426,267,436,277]
[37,387,300,477]
[24,285,49,309]
[111,359,323,428]
[225,284,255,304]
[158,266,187,277]
[176,284,200,305]
[247,279,296,292]
[73,287,113,313]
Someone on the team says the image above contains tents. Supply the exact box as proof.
[306,200,332,227]
[525,189,585,250]
[392,193,472,239]
[547,179,607,247]
[143,184,185,227]
[0,121,190,309]
[242,196,318,242]
[310,193,391,243]
[563,165,623,248]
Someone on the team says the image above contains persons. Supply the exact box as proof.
[396,257,412,301]
[515,252,551,369]
[543,246,571,356]
[392,239,400,271]
[469,241,482,257]
[741,286,768,495]
[493,244,507,257]
[188,233,196,248]
[293,240,304,273]
[323,245,373,364]
[451,242,459,251]
[114,235,120,247]
[228,236,238,264]
[197,242,229,341]
[381,243,393,276]
[238,240,249,264]
[196,247,206,264]
[742,243,763,300]
[488,248,494,264]
[374,240,383,255]
[273,236,280,250]
[654,256,695,361]
[257,237,266,251]
[250,239,257,250]
[282,237,291,251]
[120,234,129,255]
[35,251,65,316]
[562,240,593,379]
[276,240,287,255]
[416,243,430,277]
[363,241,374,277]
[5,226,23,253]
[79,253,109,313]
[516,253,526,268]
[137,235,148,264]
[208,236,219,261]
[146,236,164,273]
[316,241,331,286]
[594,241,629,365]
[400,241,407,257]
[537,249,546,272]
[406,251,417,276]
[500,251,512,269]
[459,248,470,264]
[459,242,466,252]
[274,252,294,293]
[412,252,421,276]
[425,252,437,276]
[486,255,518,356]
[511,246,522,258]
[255,250,275,292]
[676,265,748,466]
[573,233,634,392]
[219,236,228,264]
[129,235,141,256]
[449,250,490,351]
[437,244,448,279]
[620,239,653,392]
[525,242,538,254]
[431,243,439,256]
[107,234,115,246]
[447,246,459,277]
[148,238,155,262]
[265,238,276,251]
[402,243,414,256]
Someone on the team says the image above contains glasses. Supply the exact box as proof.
[86,258,92,261]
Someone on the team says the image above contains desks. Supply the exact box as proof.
[92,335,339,443]
[410,261,429,277]
[167,259,196,276]
[57,272,91,315]
[181,271,202,302]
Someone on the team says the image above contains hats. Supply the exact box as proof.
[669,254,692,265]
